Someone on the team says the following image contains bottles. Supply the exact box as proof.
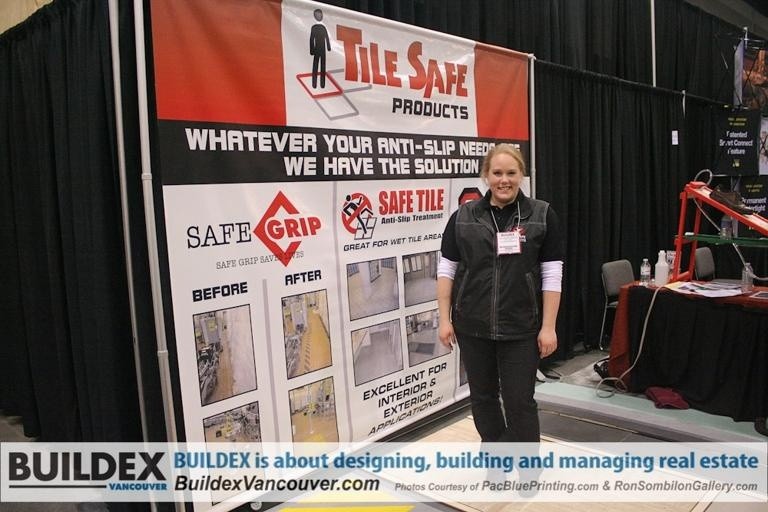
[720,214,732,241]
[639,251,670,288]
[741,263,753,293]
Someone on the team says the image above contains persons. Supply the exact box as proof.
[434,144,564,497]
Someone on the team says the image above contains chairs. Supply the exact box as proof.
[694,246,716,281]
[598,259,638,351]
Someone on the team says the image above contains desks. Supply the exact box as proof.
[611,278,767,419]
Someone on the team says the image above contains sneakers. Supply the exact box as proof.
[517,473,540,498]
[484,465,507,492]
